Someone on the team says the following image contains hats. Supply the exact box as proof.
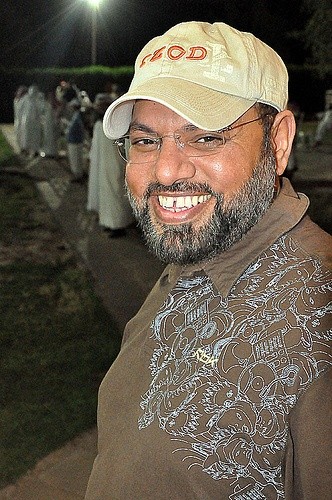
[102,21,289,140]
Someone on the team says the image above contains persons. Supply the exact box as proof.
[84,20,332,500]
[10,78,137,240]
[282,86,332,181]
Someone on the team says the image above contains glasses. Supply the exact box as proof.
[112,113,266,164]
